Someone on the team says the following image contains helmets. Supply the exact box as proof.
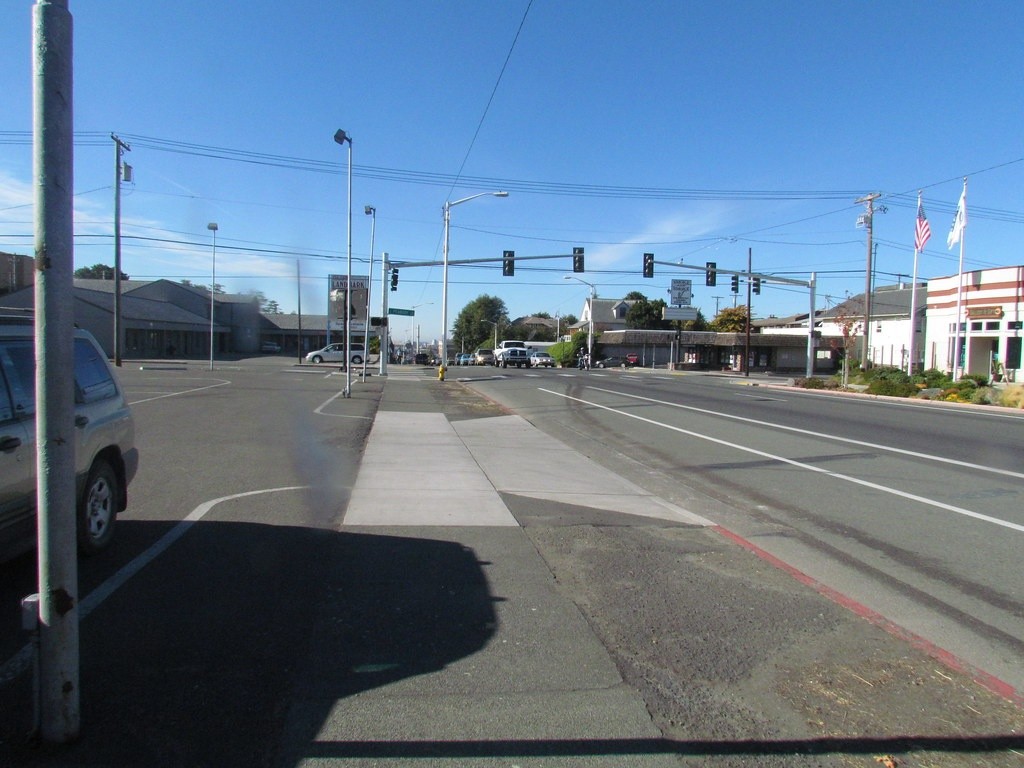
[581,347,585,351]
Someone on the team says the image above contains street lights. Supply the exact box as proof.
[208,223,218,371]
[442,190,509,365]
[363,205,376,384]
[412,302,434,353]
[564,277,593,369]
[481,320,497,348]
[333,129,352,398]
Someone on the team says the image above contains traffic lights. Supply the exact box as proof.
[391,268,399,292]
[753,277,760,295]
[731,275,739,293]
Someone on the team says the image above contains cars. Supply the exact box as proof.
[531,352,555,368]
[414,353,475,366]
[475,349,494,366]
[306,343,370,364]
[596,357,629,368]
[627,353,639,366]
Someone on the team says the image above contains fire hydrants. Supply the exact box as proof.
[439,365,448,381]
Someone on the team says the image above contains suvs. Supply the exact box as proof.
[0,305,139,671]
[494,340,533,369]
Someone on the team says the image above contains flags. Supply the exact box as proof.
[947,186,967,251]
[915,196,930,253]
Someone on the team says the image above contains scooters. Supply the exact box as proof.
[578,353,591,371]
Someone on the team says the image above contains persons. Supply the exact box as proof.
[577,347,586,367]
